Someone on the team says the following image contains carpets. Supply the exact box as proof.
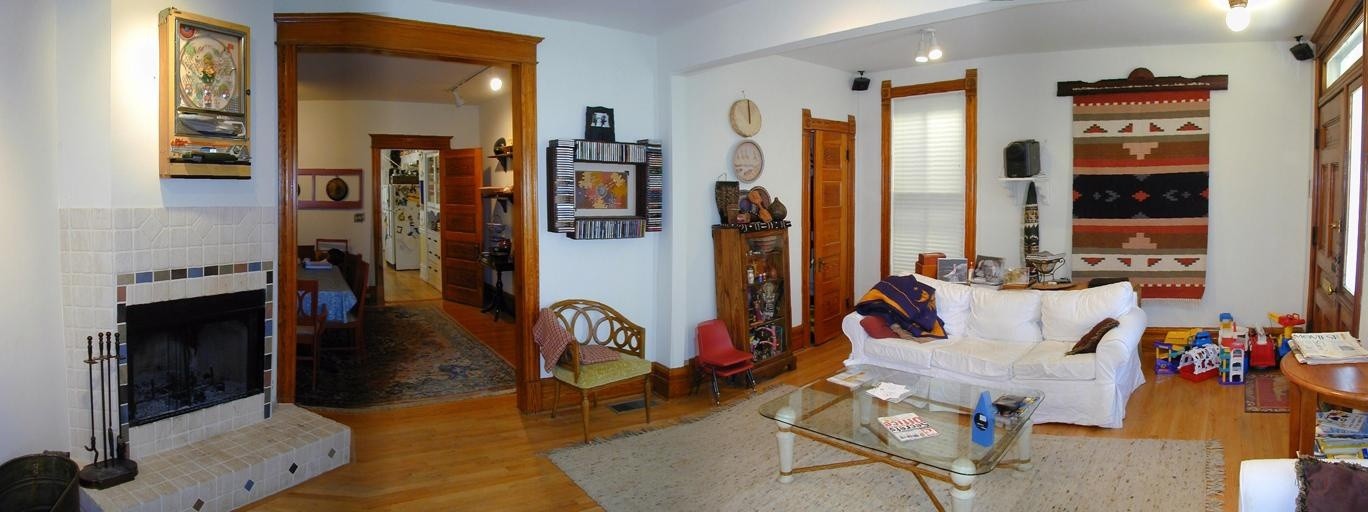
[293,302,517,417]
[543,383,1226,511]
[1242,366,1324,416]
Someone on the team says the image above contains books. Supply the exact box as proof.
[877,413,942,441]
[550,139,663,240]
[1313,406,1368,466]
[1284,329,1368,366]
[994,393,1039,419]
[868,382,915,404]
[823,368,876,388]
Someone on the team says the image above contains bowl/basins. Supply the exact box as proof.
[746,236,778,254]
[485,223,507,234]
[476,187,504,197]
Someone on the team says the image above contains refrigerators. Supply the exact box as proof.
[382,184,419,271]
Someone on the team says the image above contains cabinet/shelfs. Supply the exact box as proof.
[413,151,443,293]
[482,152,513,204]
[712,219,797,384]
[543,138,667,244]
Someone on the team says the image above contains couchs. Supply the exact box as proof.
[840,270,1147,429]
[1238,455,1367,512]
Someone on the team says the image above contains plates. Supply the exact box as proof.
[325,177,348,201]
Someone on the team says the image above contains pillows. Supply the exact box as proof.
[896,270,1134,344]
[1293,455,1368,512]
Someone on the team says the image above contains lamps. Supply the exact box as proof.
[1225,0,1251,31]
[445,65,492,108]
[914,26,942,64]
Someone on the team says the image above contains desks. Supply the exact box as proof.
[1280,349,1367,460]
[478,251,515,322]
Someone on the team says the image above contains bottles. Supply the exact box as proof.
[500,238,511,256]
[746,267,754,284]
[758,271,766,283]
[968,261,975,281]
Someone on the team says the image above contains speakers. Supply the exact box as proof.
[1290,43,1314,60]
[852,77,870,90]
[1005,141,1040,177]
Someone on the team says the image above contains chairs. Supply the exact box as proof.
[544,298,654,445]
[296,279,332,390]
[690,320,761,404]
[324,249,370,366]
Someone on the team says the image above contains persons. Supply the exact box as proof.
[592,114,598,125]
[600,115,607,126]
